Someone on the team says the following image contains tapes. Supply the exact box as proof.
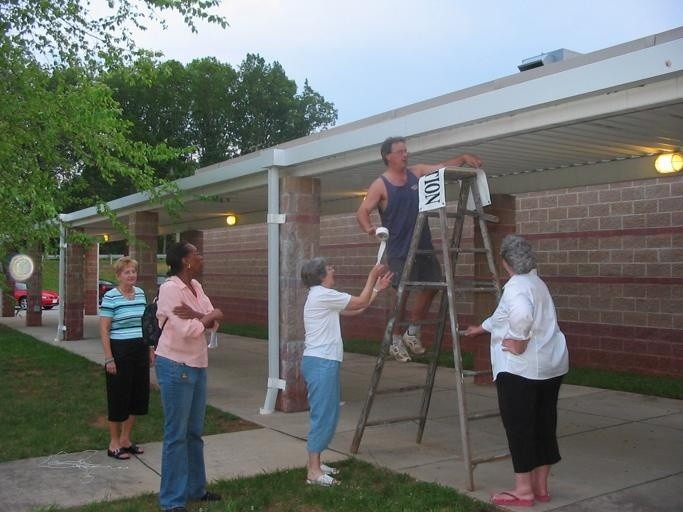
[375,227,389,265]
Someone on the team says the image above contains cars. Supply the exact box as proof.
[156,273,166,289]
[14,281,57,309]
[98,277,115,304]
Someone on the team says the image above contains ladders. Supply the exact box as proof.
[349,167,512,492]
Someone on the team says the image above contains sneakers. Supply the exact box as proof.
[388,329,427,363]
[200,491,222,502]
[165,506,187,512]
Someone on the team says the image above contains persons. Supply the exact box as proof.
[298,253,396,489]
[353,135,484,363]
[100,255,155,460]
[465,233,570,506]
[151,241,228,512]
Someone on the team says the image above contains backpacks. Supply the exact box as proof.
[141,279,180,347]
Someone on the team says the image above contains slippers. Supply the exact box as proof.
[304,463,342,487]
[489,490,550,507]
[108,442,144,459]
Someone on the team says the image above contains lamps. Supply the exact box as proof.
[226,215,236,226]
[654,152,683,175]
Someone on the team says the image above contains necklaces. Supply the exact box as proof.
[118,288,133,295]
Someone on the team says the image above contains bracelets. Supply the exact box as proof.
[372,287,381,294]
[103,356,114,366]
